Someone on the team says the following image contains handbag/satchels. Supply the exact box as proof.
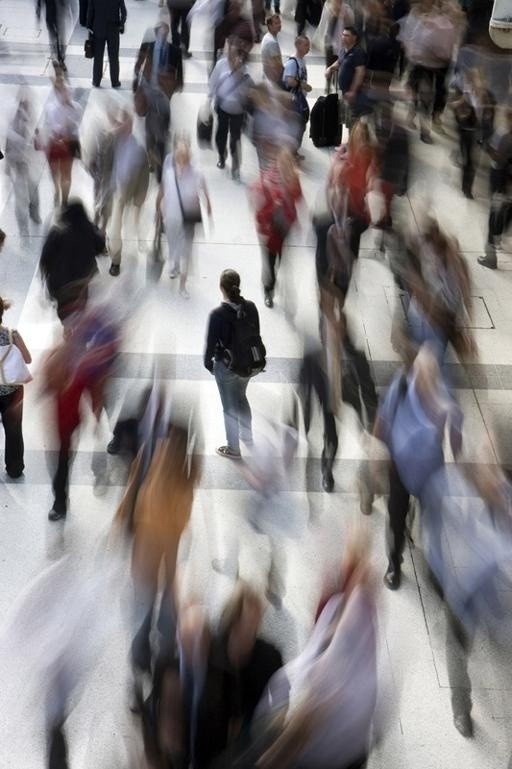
[293,91,309,124]
[0,343,33,386]
[85,32,94,58]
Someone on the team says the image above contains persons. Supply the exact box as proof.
[1,4,511,769]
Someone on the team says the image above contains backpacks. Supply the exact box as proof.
[214,307,267,377]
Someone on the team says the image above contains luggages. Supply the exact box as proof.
[310,69,342,147]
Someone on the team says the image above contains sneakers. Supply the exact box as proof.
[215,446,242,460]
[48,510,62,520]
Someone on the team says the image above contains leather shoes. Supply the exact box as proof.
[110,263,120,276]
[478,256,496,269]
[323,478,334,493]
[384,571,399,590]
[265,297,273,307]
[454,714,473,737]
